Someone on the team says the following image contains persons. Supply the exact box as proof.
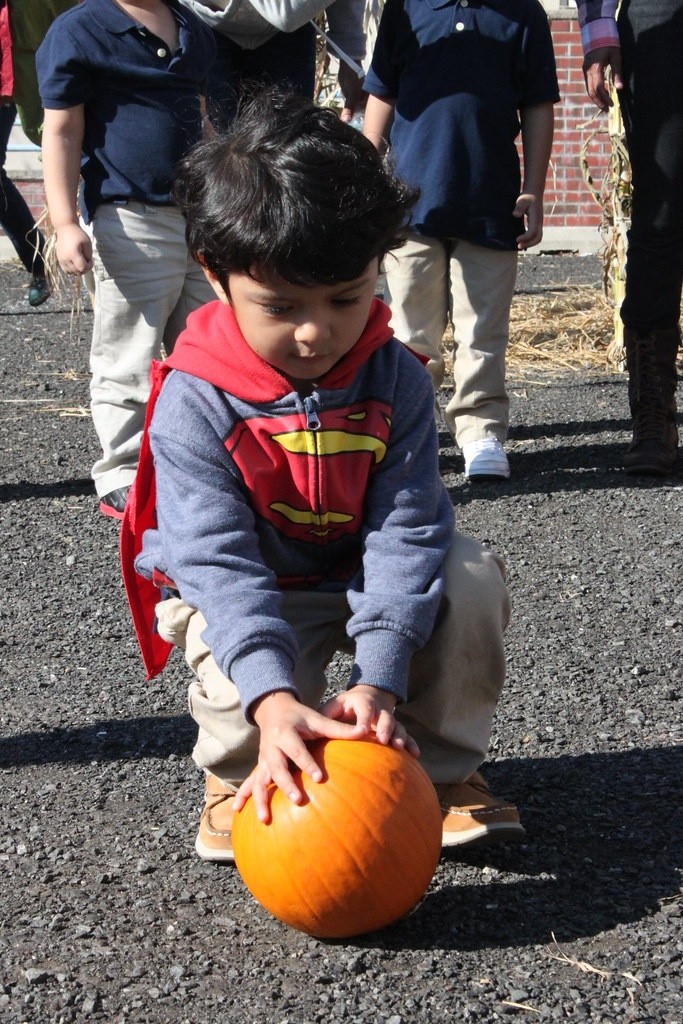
[34,0,234,518]
[0,95,57,307]
[204,0,369,135]
[576,0,683,474]
[135,89,526,865]
[361,0,560,480]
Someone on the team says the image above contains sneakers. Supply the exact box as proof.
[432,772,526,847]
[195,795,236,862]
[29,277,53,305]
[99,485,132,521]
[462,436,510,480]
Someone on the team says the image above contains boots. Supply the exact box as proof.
[623,327,678,473]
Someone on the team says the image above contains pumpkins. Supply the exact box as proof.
[231,730,443,937]
